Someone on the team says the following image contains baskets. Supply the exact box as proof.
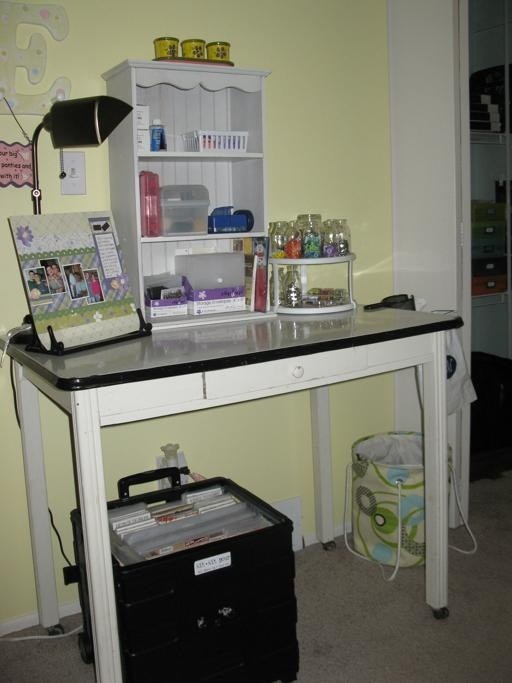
[182,129,249,152]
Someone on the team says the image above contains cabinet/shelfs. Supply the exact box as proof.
[469,0,512,308]
[101,58,278,330]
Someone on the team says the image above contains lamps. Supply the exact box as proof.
[10,95,134,345]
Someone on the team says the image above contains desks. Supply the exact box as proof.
[0,305,464,683]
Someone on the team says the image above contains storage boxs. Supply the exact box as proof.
[470,199,507,296]
[159,185,210,236]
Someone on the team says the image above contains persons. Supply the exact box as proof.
[32,271,49,294]
[90,271,101,302]
[83,270,95,303]
[50,264,61,279]
[27,270,37,291]
[73,271,87,296]
[45,265,63,292]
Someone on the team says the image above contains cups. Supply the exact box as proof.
[153,37,231,61]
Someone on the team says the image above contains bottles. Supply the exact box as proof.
[269,265,304,308]
[268,214,350,259]
[150,119,165,151]
